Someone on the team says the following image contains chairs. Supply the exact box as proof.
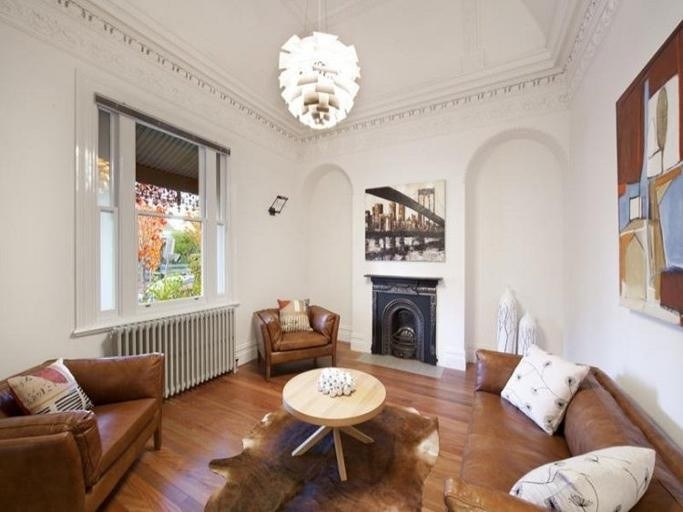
[254,305,340,382]
[0,352,165,512]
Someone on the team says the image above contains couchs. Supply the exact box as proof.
[442,349,683,512]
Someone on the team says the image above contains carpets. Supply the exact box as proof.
[203,402,440,511]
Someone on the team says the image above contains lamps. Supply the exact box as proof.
[277,0,362,129]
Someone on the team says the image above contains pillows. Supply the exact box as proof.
[5,358,96,413]
[277,298,314,333]
[500,344,590,437]
[508,446,656,511]
[0,393,25,415]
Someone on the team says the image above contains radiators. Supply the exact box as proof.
[108,302,240,400]
